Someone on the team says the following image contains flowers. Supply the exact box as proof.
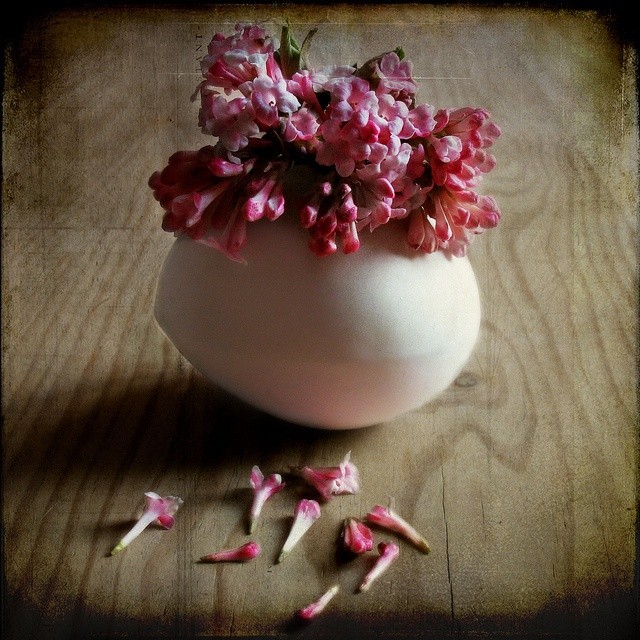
[146,24,500,254]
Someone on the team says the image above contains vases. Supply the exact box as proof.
[152,197,483,432]
